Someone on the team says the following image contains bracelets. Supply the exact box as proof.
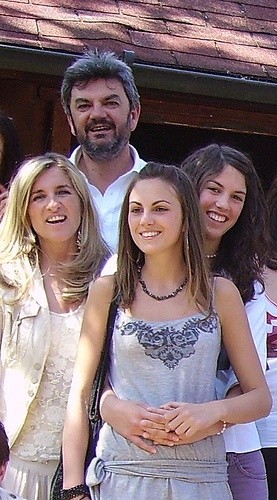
[217,420,227,436]
[58,484,92,500]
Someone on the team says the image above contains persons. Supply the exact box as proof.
[0,153,117,500]
[59,163,273,500]
[0,421,25,499]
[102,143,269,500]
[59,50,150,255]
[0,111,22,219]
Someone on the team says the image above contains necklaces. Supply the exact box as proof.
[138,263,188,301]
[201,251,223,258]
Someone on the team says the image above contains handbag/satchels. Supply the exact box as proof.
[49,418,94,500]
